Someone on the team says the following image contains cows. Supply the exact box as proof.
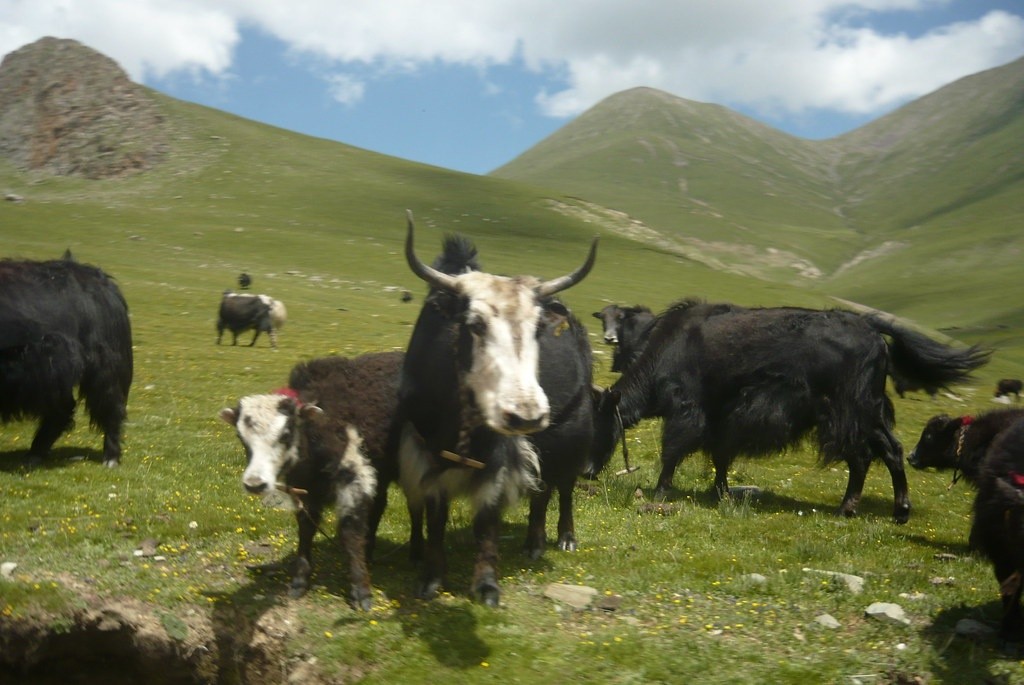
[907,379,1024,660]
[0,248,134,468]
[215,288,287,347]
[221,209,993,609]
